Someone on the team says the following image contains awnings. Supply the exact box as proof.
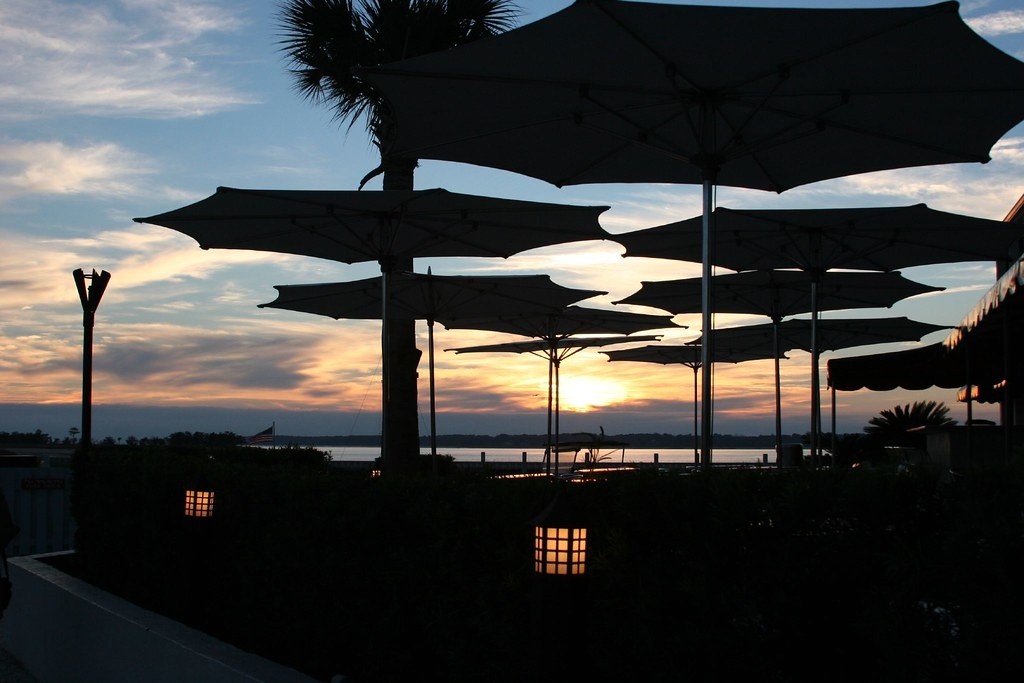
[942,254,1024,352]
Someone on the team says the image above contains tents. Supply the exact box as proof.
[827,343,974,463]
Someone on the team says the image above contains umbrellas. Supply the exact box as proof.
[447,335,663,478]
[611,202,1024,471]
[439,304,680,476]
[687,318,955,471]
[367,0,1024,467]
[134,188,616,466]
[260,272,609,458]
[607,344,788,469]
[611,266,945,468]
